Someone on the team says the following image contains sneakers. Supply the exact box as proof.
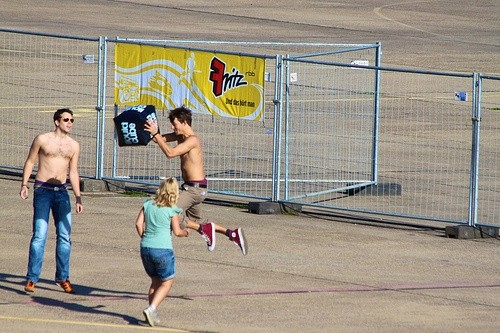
[55,280,74,293]
[229,226,248,256]
[24,279,34,294]
[199,222,216,252]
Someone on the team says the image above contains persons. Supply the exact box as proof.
[135,177,190,327]
[20,108,84,293]
[144,104,249,256]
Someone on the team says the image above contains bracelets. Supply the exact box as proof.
[21,184,30,189]
[184,230,188,237]
[153,133,158,137]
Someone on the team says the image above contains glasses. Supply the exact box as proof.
[64,118,74,123]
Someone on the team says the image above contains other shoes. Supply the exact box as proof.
[143,305,160,327]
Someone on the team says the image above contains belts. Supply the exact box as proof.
[183,181,207,188]
[34,184,67,191]
